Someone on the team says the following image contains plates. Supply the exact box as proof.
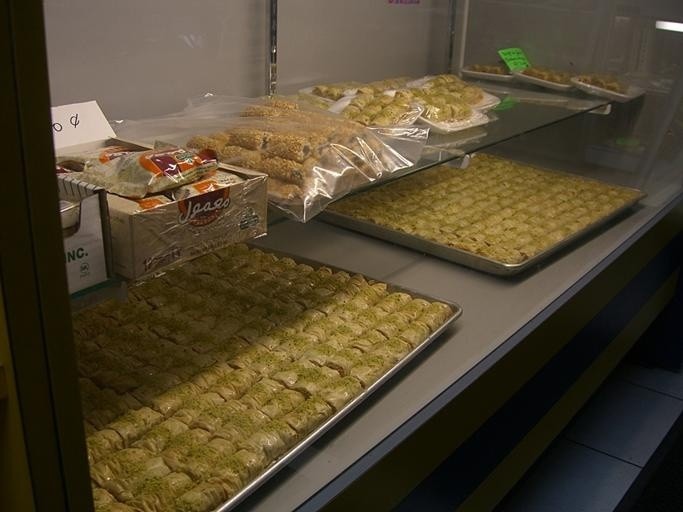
[571,69,646,103]
[517,64,573,91]
[462,64,512,84]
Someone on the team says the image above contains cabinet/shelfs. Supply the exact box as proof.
[0,0,682,512]
